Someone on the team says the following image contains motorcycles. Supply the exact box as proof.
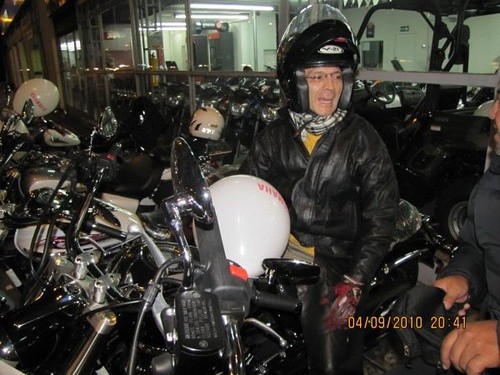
[0,0,500,375]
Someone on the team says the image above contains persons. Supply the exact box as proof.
[391,85,500,375]
[235,19,400,374]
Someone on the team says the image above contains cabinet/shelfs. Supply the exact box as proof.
[193,32,234,72]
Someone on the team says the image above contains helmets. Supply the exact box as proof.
[209,174,290,278]
[277,4,360,114]
[13,78,60,117]
[189,107,225,140]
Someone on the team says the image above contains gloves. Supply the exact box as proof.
[320,275,364,335]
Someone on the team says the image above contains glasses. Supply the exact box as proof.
[306,72,342,81]
